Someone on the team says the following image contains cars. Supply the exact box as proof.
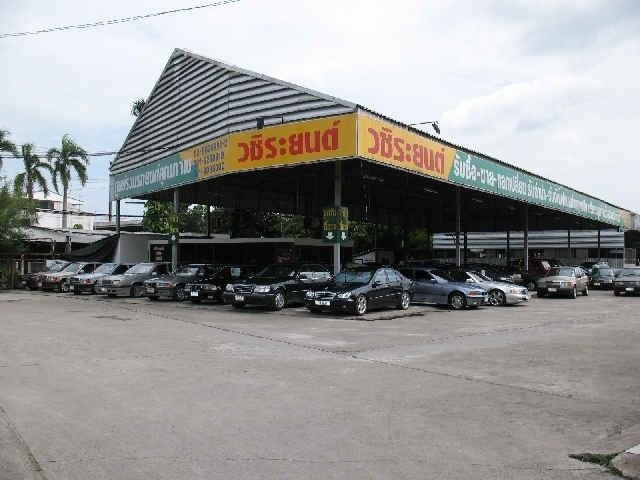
[68,261,139,296]
[182,265,256,305]
[103,261,173,298]
[20,263,66,289]
[463,269,531,308]
[143,262,224,302]
[611,265,640,296]
[222,261,331,313]
[398,265,489,312]
[467,261,529,285]
[302,262,415,317]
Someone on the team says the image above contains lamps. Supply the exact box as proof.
[410,121,441,134]
[256,115,284,128]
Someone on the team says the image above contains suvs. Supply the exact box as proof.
[41,261,102,294]
[535,266,590,300]
[588,258,616,290]
